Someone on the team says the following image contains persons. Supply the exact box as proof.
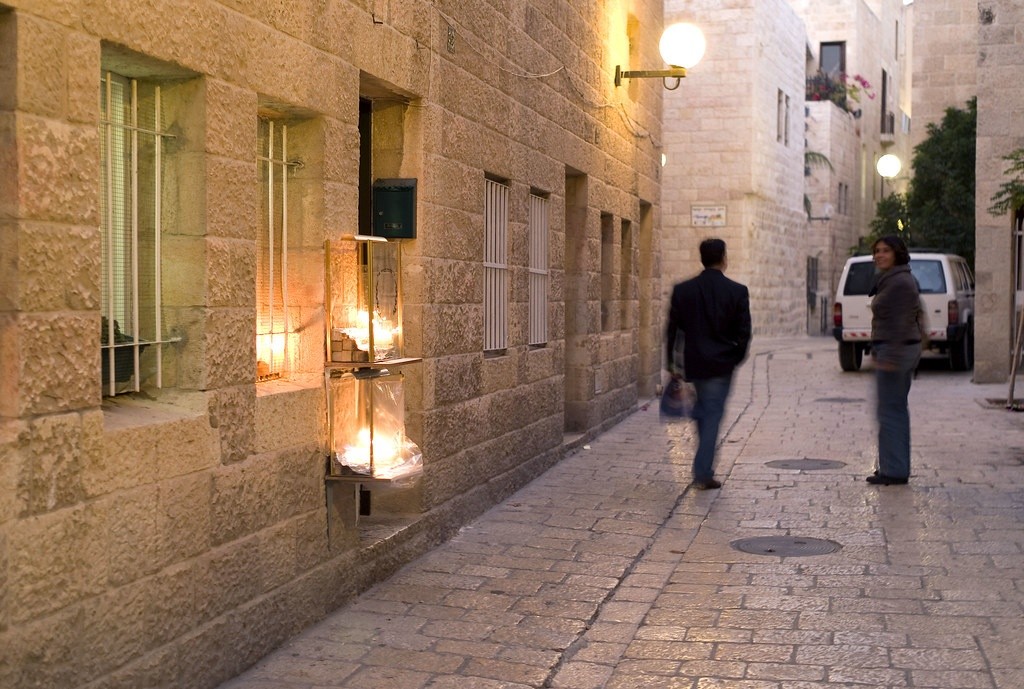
[664,239,753,489]
[866,236,924,485]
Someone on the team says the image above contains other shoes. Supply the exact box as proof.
[866,470,908,485]
[698,479,721,489]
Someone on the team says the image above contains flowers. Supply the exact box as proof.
[806,68,876,112]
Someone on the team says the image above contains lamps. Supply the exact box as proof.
[616,23,704,91]
[876,154,907,187]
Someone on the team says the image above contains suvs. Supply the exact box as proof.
[831,251,974,371]
